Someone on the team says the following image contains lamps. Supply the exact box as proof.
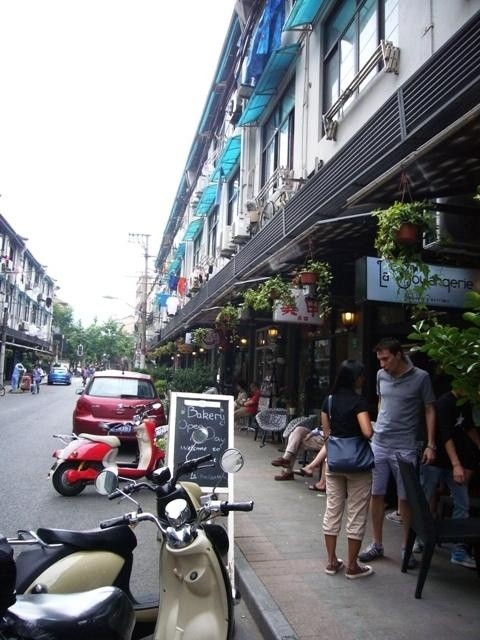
[267,322,278,338]
[341,302,356,333]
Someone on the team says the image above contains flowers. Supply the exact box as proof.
[275,384,298,408]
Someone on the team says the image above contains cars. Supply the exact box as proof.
[70,368,167,442]
[48,366,73,385]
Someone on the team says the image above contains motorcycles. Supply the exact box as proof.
[45,398,166,499]
[7,425,229,627]
[0,448,254,640]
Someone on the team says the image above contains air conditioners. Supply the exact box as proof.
[230,216,251,244]
[188,273,199,292]
[217,225,237,258]
[227,90,242,125]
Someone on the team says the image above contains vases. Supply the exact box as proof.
[289,407,296,414]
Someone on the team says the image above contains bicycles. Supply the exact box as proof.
[0,384,6,397]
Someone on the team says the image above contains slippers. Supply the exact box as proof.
[309,484,325,492]
[295,468,312,477]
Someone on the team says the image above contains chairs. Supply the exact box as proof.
[282,414,318,470]
[254,407,288,444]
[436,496,480,548]
[239,414,256,432]
[394,452,480,599]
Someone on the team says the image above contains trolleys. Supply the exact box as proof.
[20,373,33,393]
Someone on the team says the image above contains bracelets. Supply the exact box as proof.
[427,443,437,451]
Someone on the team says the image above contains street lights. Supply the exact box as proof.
[99,295,147,369]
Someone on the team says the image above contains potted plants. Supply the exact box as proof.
[370,200,453,322]
[219,259,334,344]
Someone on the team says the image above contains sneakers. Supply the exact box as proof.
[451,556,476,568]
[386,510,404,526]
[400,547,415,569]
[346,564,373,579]
[360,545,383,562]
[325,559,344,575]
[274,470,294,481]
[271,457,291,468]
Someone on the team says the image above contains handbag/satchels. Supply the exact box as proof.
[328,435,375,471]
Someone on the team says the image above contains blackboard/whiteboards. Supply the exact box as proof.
[174,397,228,488]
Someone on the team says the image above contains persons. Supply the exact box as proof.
[82,359,111,385]
[291,438,328,491]
[356,336,438,569]
[321,358,374,578]
[385,510,403,524]
[31,364,42,394]
[412,381,480,569]
[234,382,261,420]
[271,425,326,481]
[11,363,26,392]
[234,380,248,411]
